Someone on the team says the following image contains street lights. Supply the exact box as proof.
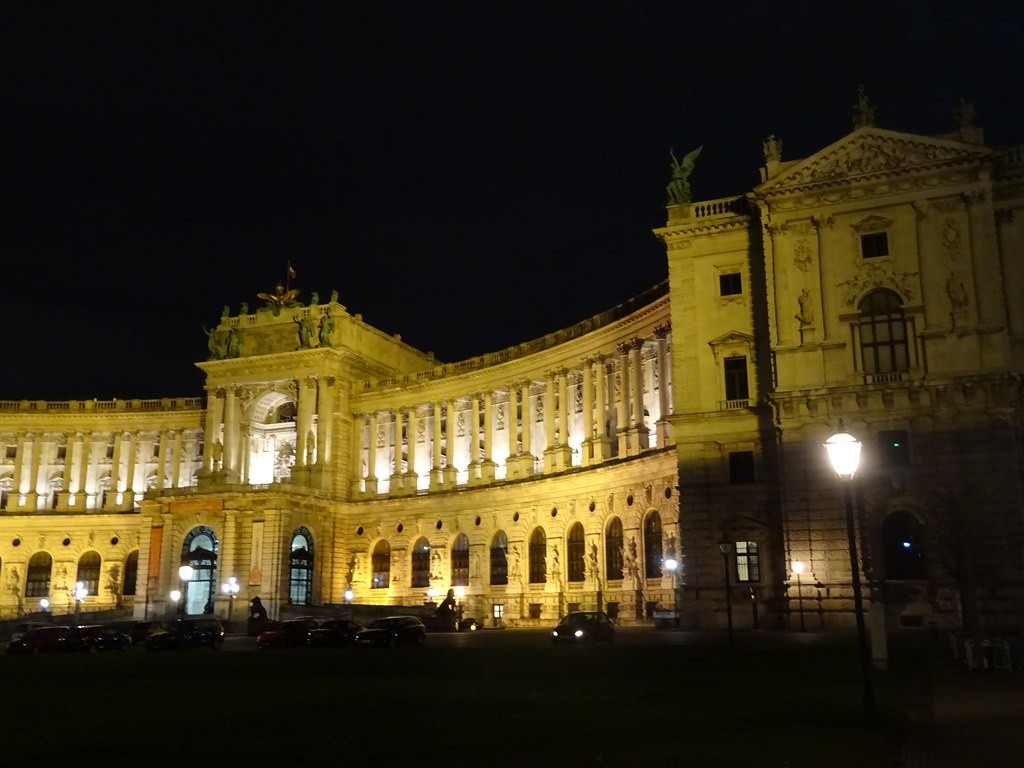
[170,590,181,621]
[70,581,88,623]
[823,417,865,651]
[345,588,353,604]
[664,540,677,587]
[428,586,435,602]
[41,595,47,615]
[222,575,239,618]
[718,531,734,646]
[456,586,466,622]
[178,560,193,605]
[791,557,805,632]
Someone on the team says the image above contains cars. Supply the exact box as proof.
[256,619,320,649]
[145,619,225,655]
[7,626,70,655]
[553,611,616,647]
[354,617,426,648]
[62,624,134,655]
[307,620,366,647]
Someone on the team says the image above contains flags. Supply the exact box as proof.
[288,264,301,279]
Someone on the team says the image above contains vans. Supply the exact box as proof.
[12,622,57,641]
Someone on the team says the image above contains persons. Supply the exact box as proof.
[249,596,269,623]
